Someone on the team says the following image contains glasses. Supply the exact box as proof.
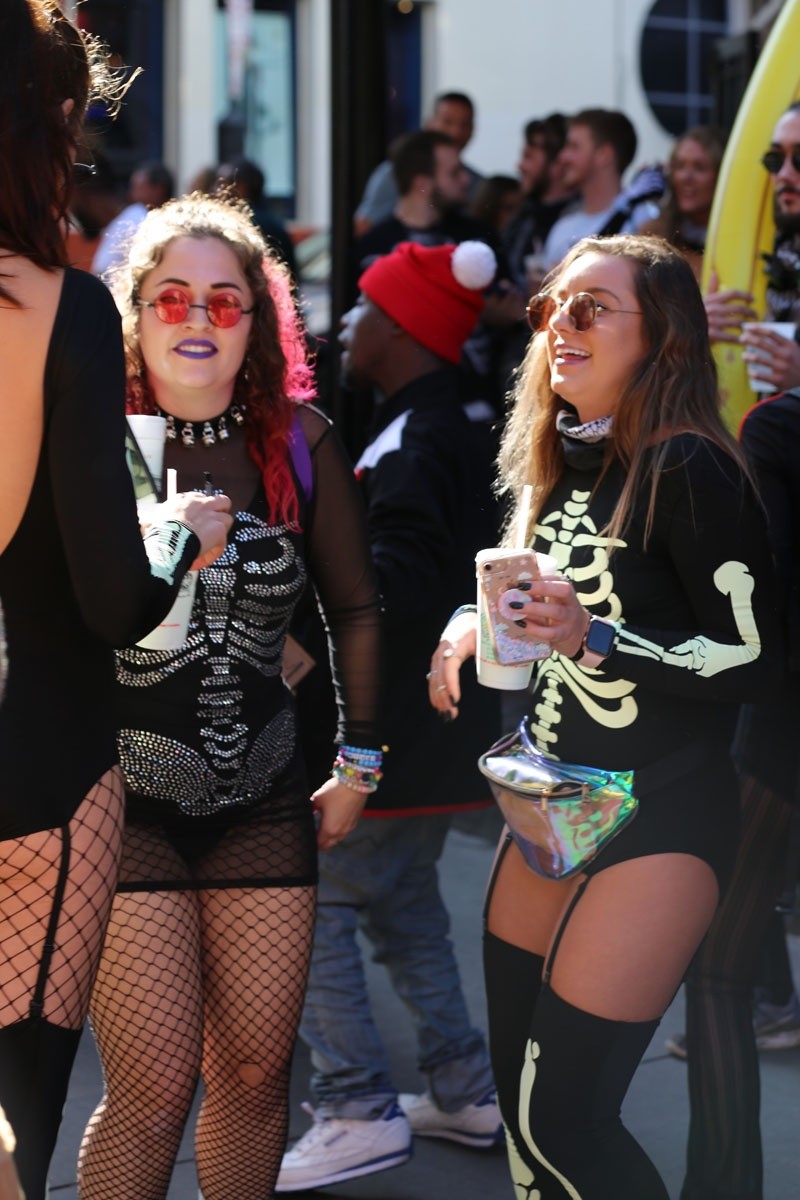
[137,288,256,329]
[526,291,647,332]
[761,150,800,173]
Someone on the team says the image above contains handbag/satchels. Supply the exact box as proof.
[477,715,729,880]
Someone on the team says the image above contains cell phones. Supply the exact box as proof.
[483,553,552,664]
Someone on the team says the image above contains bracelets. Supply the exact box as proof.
[330,746,383,792]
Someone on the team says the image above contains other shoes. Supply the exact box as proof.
[664,996,800,1059]
[450,802,506,844]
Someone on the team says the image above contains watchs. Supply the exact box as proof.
[569,614,616,668]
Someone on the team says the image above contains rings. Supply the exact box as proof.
[426,671,437,680]
[442,649,463,661]
[436,684,447,692]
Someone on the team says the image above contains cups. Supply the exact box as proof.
[130,503,198,650]
[476,551,556,690]
[740,321,797,394]
[126,412,167,495]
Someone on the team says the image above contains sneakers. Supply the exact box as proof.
[276,1100,411,1191]
[396,1091,502,1147]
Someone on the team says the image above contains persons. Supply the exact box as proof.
[666,385,799,1059]
[272,241,504,1193]
[0,2,232,1200]
[426,233,799,1200]
[76,192,388,1200]
[0,1104,25,1200]
[56,92,800,410]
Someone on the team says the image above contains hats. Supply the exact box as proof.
[359,241,497,364]
